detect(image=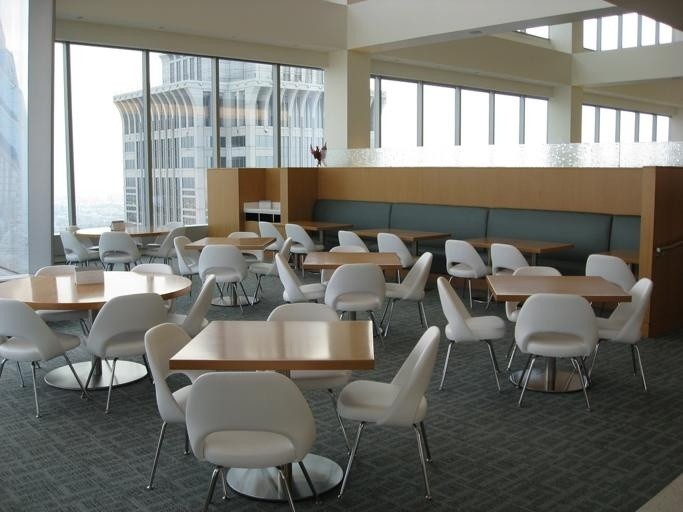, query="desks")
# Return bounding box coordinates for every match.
[241,200,281,223]
